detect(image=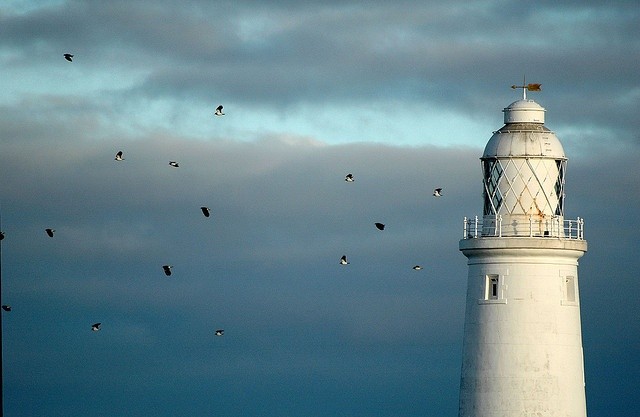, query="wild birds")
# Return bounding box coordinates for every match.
[344,172,357,184]
[214,105,226,118]
[62,52,75,63]
[214,329,226,338]
[114,150,125,163]
[162,264,174,276]
[44,228,56,239]
[1,231,6,241]
[1,304,13,313]
[432,187,444,200]
[199,206,212,219]
[412,265,424,272]
[338,254,349,267]
[167,160,180,168]
[91,322,102,333]
[373,221,387,233]
